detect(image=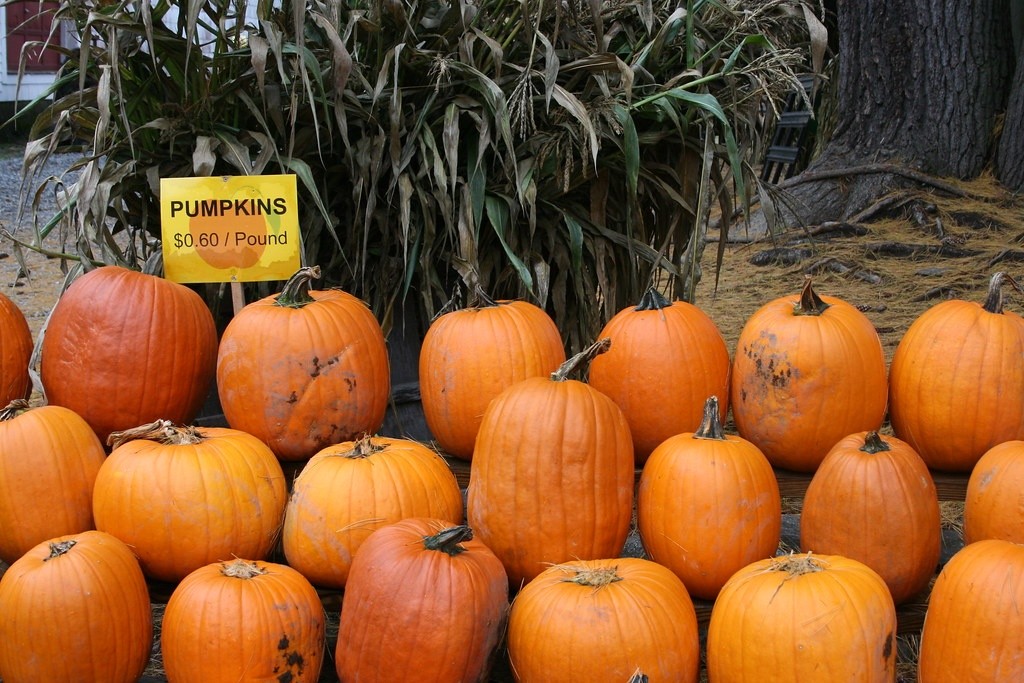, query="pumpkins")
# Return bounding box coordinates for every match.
[0,250,1024,683]
